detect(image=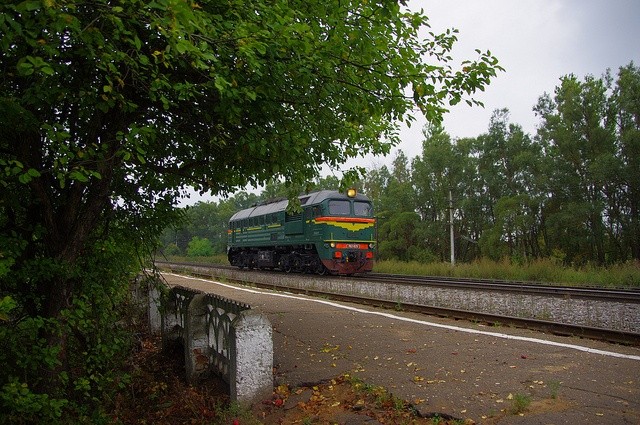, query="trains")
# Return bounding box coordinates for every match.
[227,188,377,276]
